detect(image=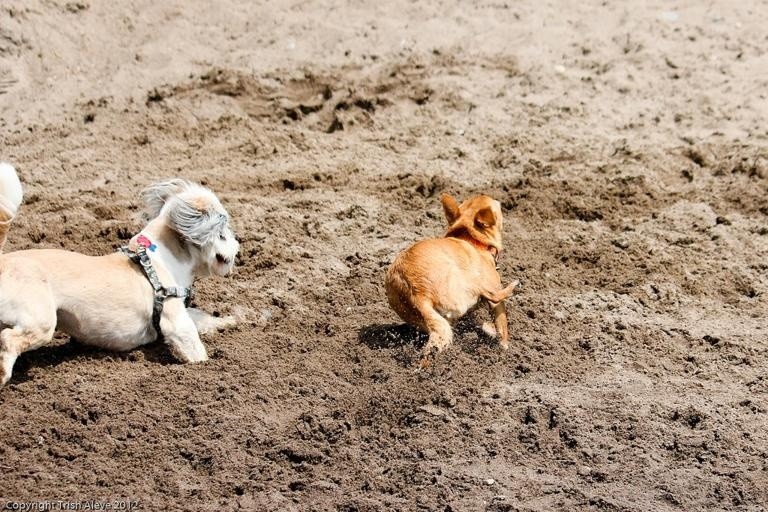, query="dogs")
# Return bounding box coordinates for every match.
[0,162,241,391]
[384,194,520,369]
